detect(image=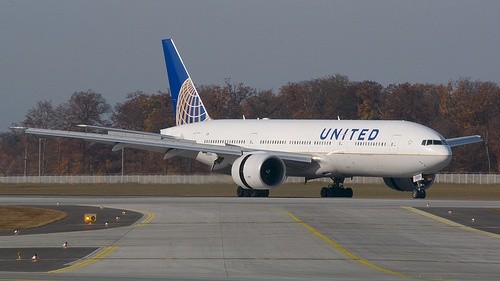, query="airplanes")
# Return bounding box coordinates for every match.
[7,38,485,198]
